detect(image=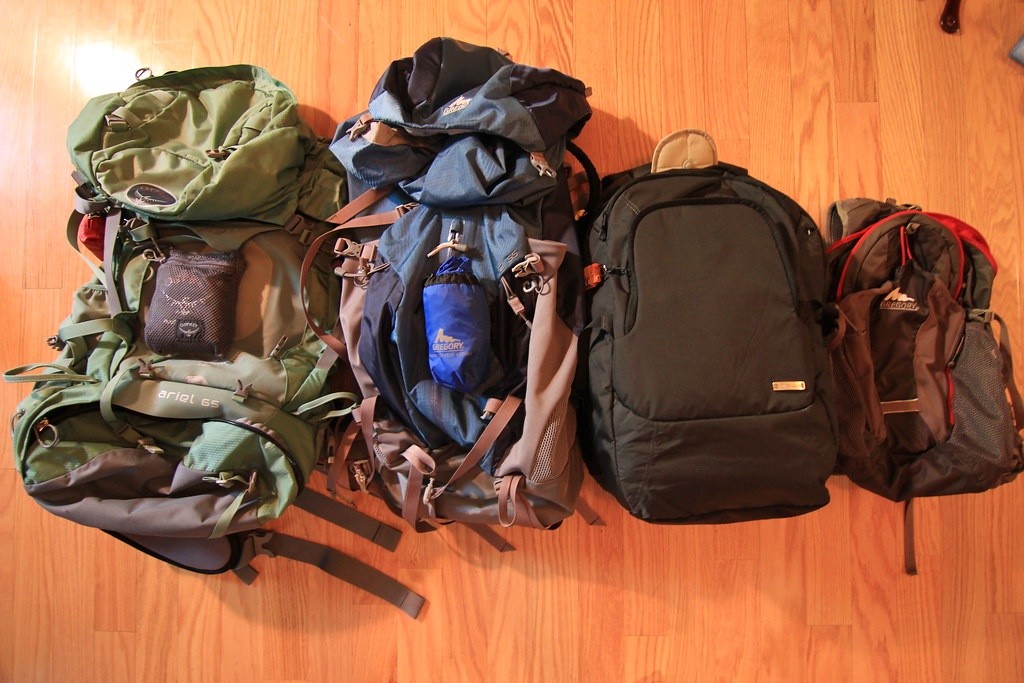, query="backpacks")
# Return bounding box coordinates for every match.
[299,35,585,531]
[818,197,1024,503]
[12,63,349,574]
[553,127,838,521]
